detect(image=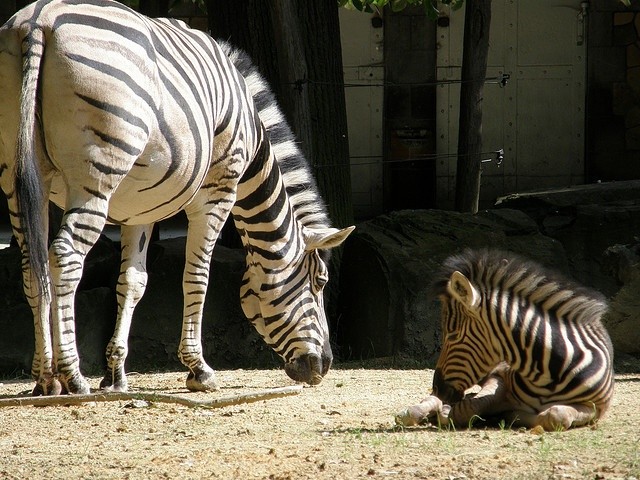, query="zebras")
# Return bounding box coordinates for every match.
[0,0,355,396]
[395,248,614,432]
